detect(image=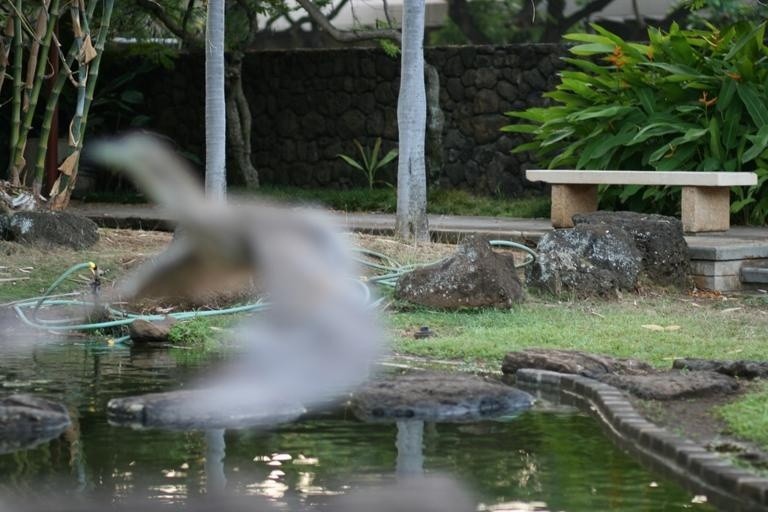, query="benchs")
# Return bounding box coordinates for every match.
[522,165,757,235]
